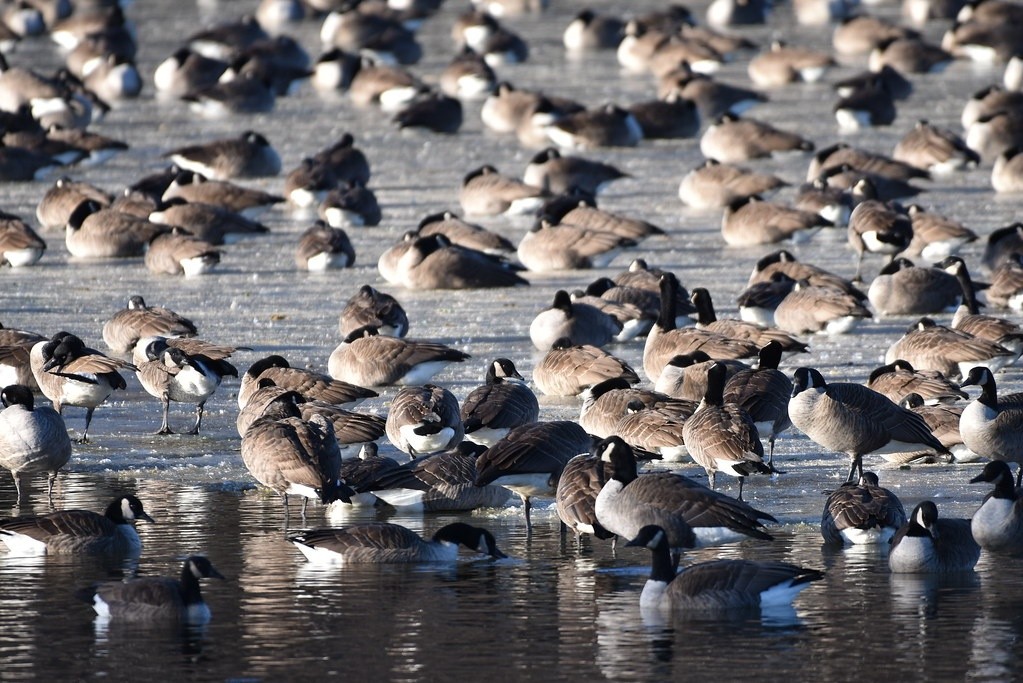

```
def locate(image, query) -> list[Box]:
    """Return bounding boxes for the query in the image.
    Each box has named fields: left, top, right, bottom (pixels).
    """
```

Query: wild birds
left=0, top=0, right=1023, bottom=619
